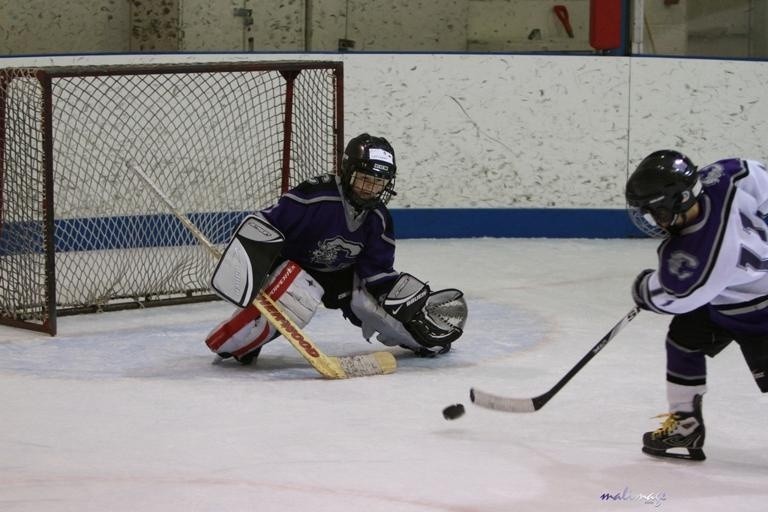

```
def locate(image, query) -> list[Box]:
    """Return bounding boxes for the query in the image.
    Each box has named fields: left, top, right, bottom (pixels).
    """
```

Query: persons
left=205, top=133, right=467, bottom=364
left=626, top=150, right=768, bottom=450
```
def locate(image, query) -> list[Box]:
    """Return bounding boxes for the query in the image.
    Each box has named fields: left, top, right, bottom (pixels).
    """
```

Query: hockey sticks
left=125, top=158, right=398, bottom=379
left=470, top=304, right=642, bottom=413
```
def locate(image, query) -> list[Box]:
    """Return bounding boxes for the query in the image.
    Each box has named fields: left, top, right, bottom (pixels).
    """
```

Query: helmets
left=340, top=133, right=397, bottom=179
left=625, top=149, right=705, bottom=213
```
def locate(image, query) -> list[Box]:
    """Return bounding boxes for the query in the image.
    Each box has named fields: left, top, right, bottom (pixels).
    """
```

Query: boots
left=643, top=412, right=706, bottom=449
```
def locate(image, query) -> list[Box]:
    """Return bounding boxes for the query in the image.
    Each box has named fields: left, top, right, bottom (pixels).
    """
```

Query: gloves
left=382, top=271, right=468, bottom=348
